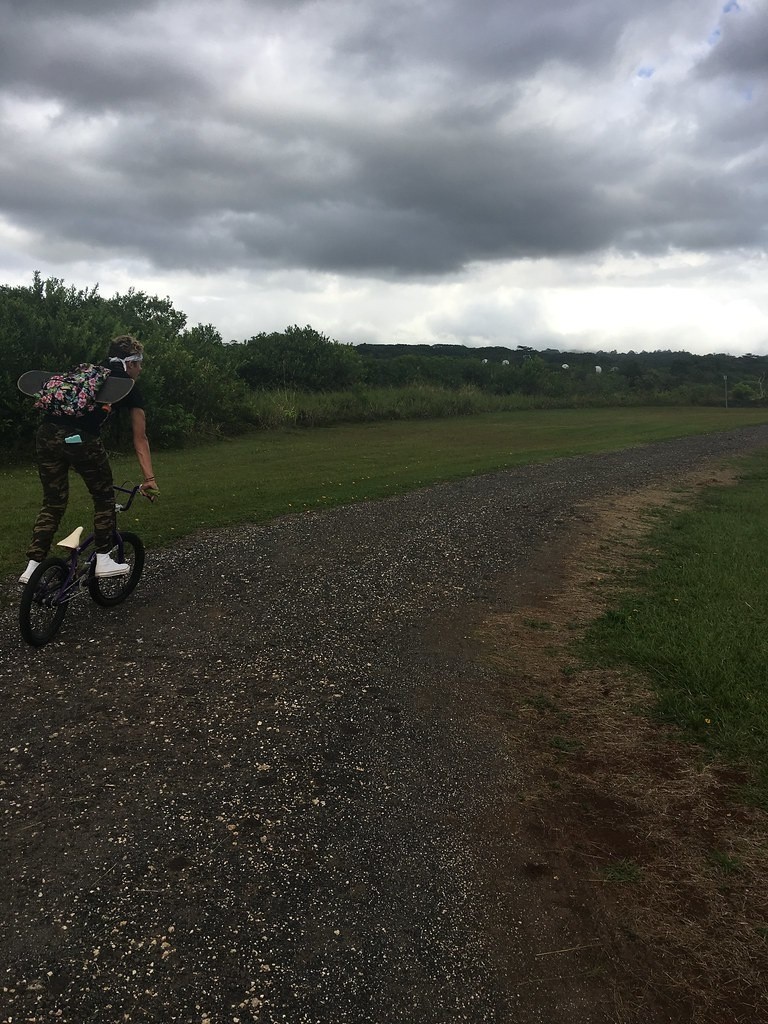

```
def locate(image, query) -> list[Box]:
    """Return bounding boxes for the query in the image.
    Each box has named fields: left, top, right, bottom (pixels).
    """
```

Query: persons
left=17, top=336, right=159, bottom=585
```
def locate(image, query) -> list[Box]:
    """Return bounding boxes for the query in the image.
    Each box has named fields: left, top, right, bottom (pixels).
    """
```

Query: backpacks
left=33, top=362, right=112, bottom=418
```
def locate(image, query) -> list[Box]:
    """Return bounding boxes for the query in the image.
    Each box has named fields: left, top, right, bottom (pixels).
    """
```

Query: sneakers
left=94, top=550, right=130, bottom=577
left=18, top=559, right=47, bottom=586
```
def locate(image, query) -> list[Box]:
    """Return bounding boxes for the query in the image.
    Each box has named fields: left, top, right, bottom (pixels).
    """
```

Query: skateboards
left=18, top=370, right=135, bottom=414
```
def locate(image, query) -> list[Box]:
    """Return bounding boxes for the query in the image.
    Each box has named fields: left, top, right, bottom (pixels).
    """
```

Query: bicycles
left=18, top=466, right=162, bottom=647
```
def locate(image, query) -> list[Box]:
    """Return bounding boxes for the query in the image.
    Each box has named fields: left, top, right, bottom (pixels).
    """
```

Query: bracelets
left=144, top=477, right=155, bottom=482
